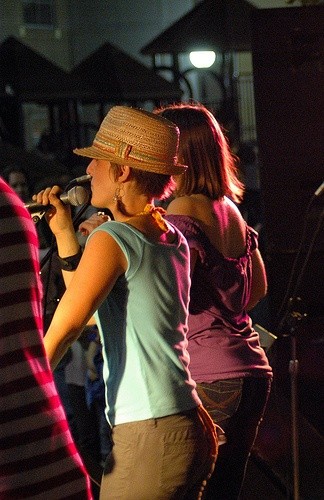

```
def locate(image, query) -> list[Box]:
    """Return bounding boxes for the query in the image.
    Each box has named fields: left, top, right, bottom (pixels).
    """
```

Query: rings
left=97, top=212, right=105, bottom=215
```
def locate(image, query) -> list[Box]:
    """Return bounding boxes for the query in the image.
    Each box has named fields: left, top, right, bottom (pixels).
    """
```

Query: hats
left=76, top=106, right=187, bottom=176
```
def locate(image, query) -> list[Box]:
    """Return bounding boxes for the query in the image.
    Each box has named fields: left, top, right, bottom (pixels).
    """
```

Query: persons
left=0, top=107, right=218, bottom=500
left=78, top=103, right=274, bottom=500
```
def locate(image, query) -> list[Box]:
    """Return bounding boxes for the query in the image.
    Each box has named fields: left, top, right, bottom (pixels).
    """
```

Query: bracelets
left=55, top=244, right=83, bottom=272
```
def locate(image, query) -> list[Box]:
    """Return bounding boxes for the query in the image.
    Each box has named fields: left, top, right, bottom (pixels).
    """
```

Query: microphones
left=76, top=175, right=91, bottom=182
left=25, top=186, right=89, bottom=212
left=314, top=182, right=324, bottom=197
